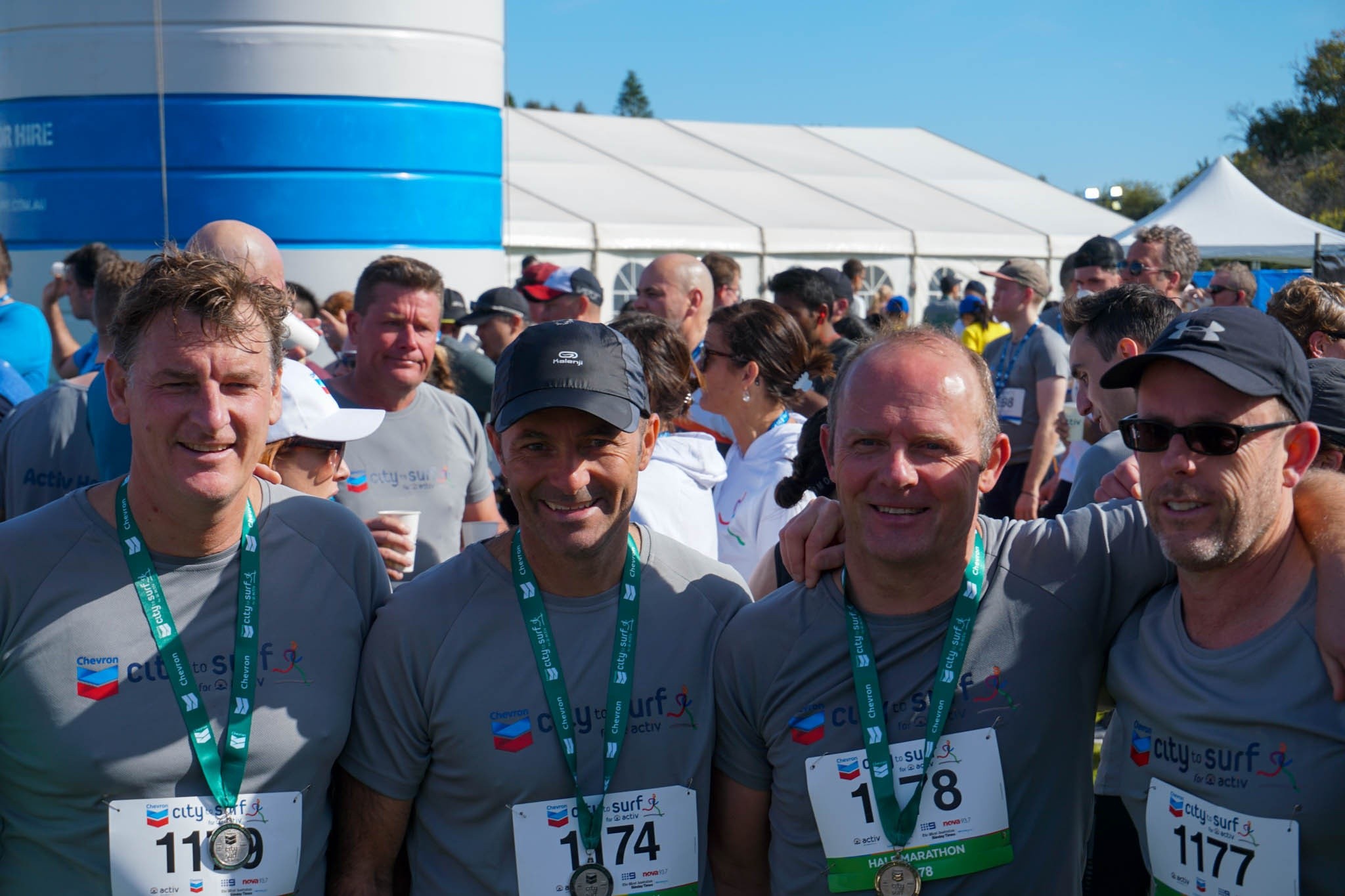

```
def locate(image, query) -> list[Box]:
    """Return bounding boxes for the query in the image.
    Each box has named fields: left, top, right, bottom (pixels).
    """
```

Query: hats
left=886, top=296, right=909, bottom=313
left=454, top=287, right=529, bottom=327
left=1072, top=236, right=1124, bottom=268
left=1097, top=306, right=1314, bottom=422
left=521, top=263, right=560, bottom=282
left=819, top=267, right=852, bottom=299
left=441, top=288, right=466, bottom=326
left=265, top=357, right=387, bottom=445
left=967, top=280, right=986, bottom=295
left=958, top=295, right=983, bottom=318
left=940, top=276, right=963, bottom=292
left=1307, top=357, right=1345, bottom=445
left=489, top=318, right=653, bottom=434
left=978, top=259, right=1051, bottom=296
left=522, top=266, right=604, bottom=306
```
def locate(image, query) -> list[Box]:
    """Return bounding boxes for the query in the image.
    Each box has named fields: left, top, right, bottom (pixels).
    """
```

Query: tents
left=1113, top=154, right=1345, bottom=267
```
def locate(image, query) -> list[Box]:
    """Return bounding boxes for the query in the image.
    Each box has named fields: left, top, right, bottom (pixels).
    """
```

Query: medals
left=875, top=847, right=921, bottom=896
left=210, top=807, right=253, bottom=870
left=570, top=848, right=614, bottom=896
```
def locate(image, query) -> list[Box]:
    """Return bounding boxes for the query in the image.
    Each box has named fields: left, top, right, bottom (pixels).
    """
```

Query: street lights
left=1110, top=185, right=1123, bottom=214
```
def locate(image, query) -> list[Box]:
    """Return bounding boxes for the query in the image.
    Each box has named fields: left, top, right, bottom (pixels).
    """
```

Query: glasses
left=726, top=286, right=741, bottom=299
left=1117, top=412, right=1300, bottom=457
left=699, top=346, right=760, bottom=387
left=1116, top=260, right=1173, bottom=277
left=1209, top=284, right=1226, bottom=294
left=469, top=301, right=524, bottom=317
left=286, top=441, right=346, bottom=472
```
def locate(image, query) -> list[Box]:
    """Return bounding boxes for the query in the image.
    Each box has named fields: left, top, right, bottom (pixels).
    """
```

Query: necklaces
left=0, top=293, right=10, bottom=303
left=767, top=409, right=789, bottom=431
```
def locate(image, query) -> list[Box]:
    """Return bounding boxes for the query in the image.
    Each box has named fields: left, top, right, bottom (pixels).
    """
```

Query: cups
left=461, top=520, right=499, bottom=548
left=279, top=308, right=321, bottom=357
left=459, top=332, right=480, bottom=352
left=1061, top=402, right=1085, bottom=442
left=378, top=510, right=422, bottom=573
left=1185, top=295, right=1215, bottom=313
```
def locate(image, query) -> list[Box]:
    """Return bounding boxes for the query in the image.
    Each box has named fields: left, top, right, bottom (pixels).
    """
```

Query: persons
left=779, top=307, right=1345, bottom=896
left=712, top=325, right=1345, bottom=896
left=44, top=241, right=121, bottom=380
left=0, top=241, right=394, bottom=895
left=328, top=318, right=756, bottom=896
left=85, top=220, right=323, bottom=482
left=0, top=238, right=52, bottom=416
left=0, top=260, right=150, bottom=515
left=288, top=224, right=1345, bottom=582
left=253, top=357, right=386, bottom=498
